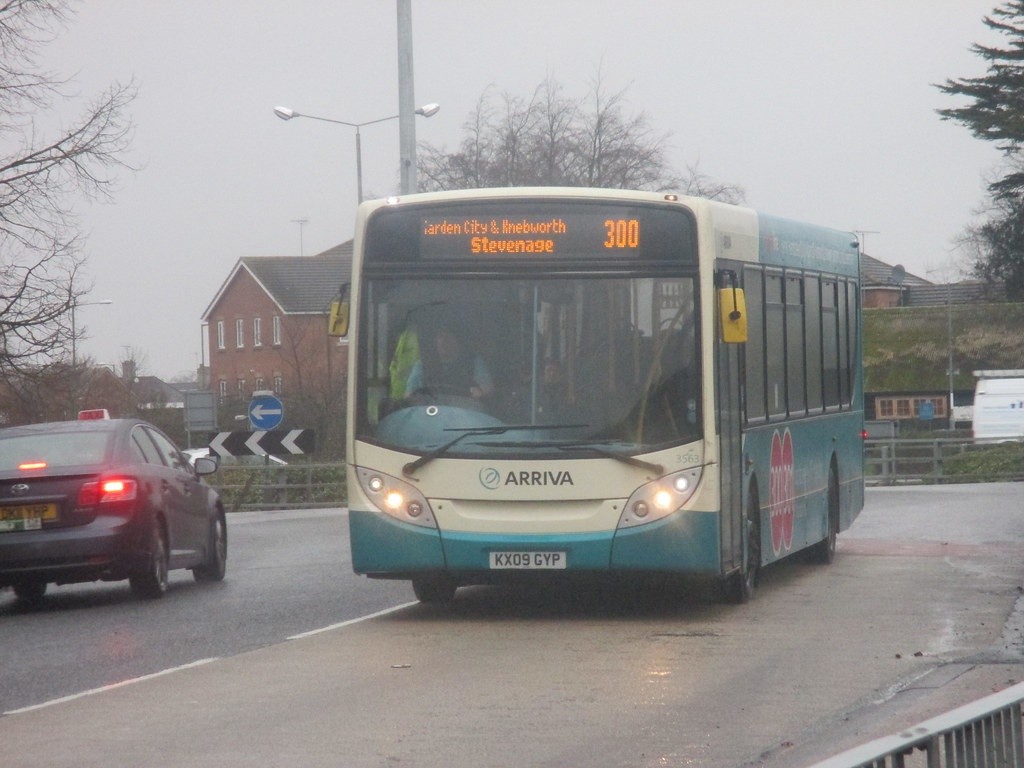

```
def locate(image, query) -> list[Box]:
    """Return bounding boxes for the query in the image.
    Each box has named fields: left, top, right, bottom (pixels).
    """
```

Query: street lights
left=272, top=102, right=441, bottom=203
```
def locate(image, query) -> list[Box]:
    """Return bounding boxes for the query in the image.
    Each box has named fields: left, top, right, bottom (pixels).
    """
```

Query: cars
left=0, top=416, right=230, bottom=604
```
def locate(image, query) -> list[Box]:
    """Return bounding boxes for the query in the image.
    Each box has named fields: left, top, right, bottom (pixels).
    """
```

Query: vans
left=972, top=376, right=1024, bottom=443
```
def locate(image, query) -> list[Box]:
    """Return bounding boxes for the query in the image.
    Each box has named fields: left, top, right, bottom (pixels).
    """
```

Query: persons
left=404, top=327, right=494, bottom=402
left=543, top=357, right=568, bottom=410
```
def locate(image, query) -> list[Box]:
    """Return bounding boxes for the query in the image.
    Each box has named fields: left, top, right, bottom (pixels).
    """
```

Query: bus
left=326, top=185, right=868, bottom=606
